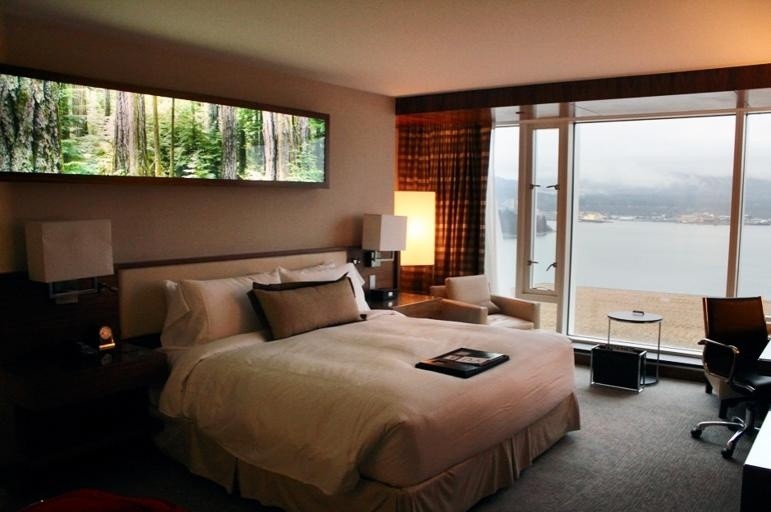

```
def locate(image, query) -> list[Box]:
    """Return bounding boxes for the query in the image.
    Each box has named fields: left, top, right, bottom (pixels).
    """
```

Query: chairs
left=430, top=285, right=541, bottom=332
left=689, top=295, right=770, bottom=457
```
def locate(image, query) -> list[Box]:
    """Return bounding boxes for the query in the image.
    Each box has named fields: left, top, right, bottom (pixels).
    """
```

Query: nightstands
left=0, top=341, right=165, bottom=511
left=367, top=288, right=442, bottom=319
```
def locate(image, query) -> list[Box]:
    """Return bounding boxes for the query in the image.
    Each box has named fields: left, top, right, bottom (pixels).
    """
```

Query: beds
left=112, top=246, right=581, bottom=511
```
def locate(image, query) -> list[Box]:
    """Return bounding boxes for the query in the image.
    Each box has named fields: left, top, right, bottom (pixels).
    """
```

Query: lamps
left=394, top=191, right=436, bottom=266
left=362, top=212, right=405, bottom=266
left=26, top=219, right=116, bottom=304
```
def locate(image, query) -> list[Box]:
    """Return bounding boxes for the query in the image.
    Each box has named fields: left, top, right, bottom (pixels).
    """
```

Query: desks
left=741, top=413, right=770, bottom=512
left=606, top=311, right=663, bottom=387
left=755, top=339, right=771, bottom=374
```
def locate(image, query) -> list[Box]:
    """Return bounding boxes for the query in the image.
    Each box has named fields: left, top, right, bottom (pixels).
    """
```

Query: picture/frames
left=0, top=63, right=330, bottom=191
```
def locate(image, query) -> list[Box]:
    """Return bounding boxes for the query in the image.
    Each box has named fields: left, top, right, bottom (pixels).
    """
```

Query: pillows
left=159, top=272, right=277, bottom=348
left=246, top=274, right=365, bottom=341
left=277, top=261, right=371, bottom=320
left=446, top=275, right=498, bottom=315
